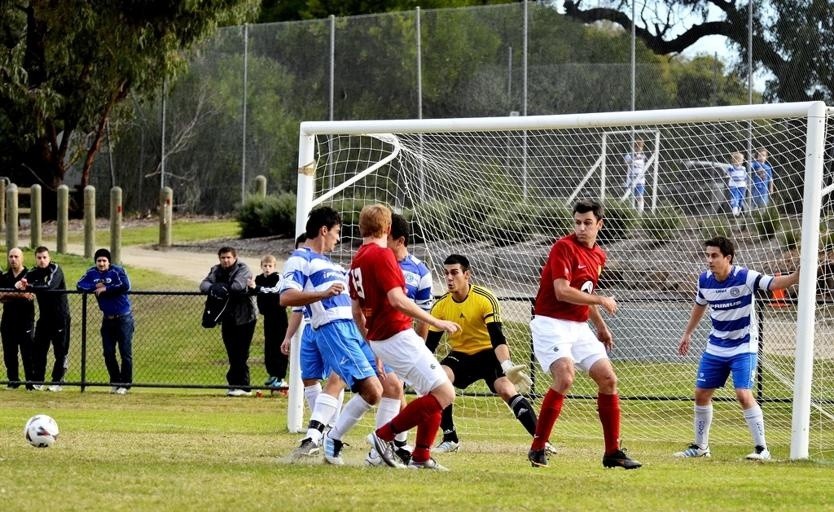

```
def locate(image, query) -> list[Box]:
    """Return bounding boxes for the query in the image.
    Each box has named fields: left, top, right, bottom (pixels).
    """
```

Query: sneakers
left=265, top=376, right=287, bottom=388
left=4, top=381, right=64, bottom=391
left=108, top=384, right=129, bottom=395
left=227, top=385, right=255, bottom=397
left=526, top=440, right=558, bottom=468
left=671, top=443, right=714, bottom=458
left=292, top=437, right=321, bottom=458
left=602, top=449, right=643, bottom=469
left=322, top=429, right=348, bottom=467
left=744, top=446, right=771, bottom=461
left=364, top=429, right=460, bottom=470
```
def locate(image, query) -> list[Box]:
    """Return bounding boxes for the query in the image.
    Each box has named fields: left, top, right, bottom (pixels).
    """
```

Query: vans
left=644, top=158, right=736, bottom=214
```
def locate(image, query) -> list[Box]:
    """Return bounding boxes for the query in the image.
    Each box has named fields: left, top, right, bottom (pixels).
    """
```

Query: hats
left=94, top=248, right=112, bottom=262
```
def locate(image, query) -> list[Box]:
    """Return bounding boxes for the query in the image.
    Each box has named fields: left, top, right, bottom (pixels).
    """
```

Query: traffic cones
left=766, top=271, right=792, bottom=307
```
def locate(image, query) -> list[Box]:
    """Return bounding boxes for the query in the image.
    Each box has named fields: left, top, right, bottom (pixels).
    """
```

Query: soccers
left=25, top=415, right=59, bottom=449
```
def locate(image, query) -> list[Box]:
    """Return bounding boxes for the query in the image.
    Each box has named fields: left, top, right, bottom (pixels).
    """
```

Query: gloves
left=498, top=358, right=533, bottom=397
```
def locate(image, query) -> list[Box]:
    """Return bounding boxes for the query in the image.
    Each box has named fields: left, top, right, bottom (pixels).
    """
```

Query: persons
left=624, top=139, right=646, bottom=216
left=76, top=249, right=135, bottom=394
left=529, top=198, right=644, bottom=469
left=14, top=246, right=72, bottom=392
left=672, top=237, right=801, bottom=459
left=424, top=254, right=556, bottom=457
left=199, top=246, right=258, bottom=397
left=725, top=153, right=747, bottom=217
left=0, top=247, right=37, bottom=391
left=278, top=201, right=464, bottom=471
left=751, top=148, right=773, bottom=216
left=247, top=255, right=289, bottom=389
